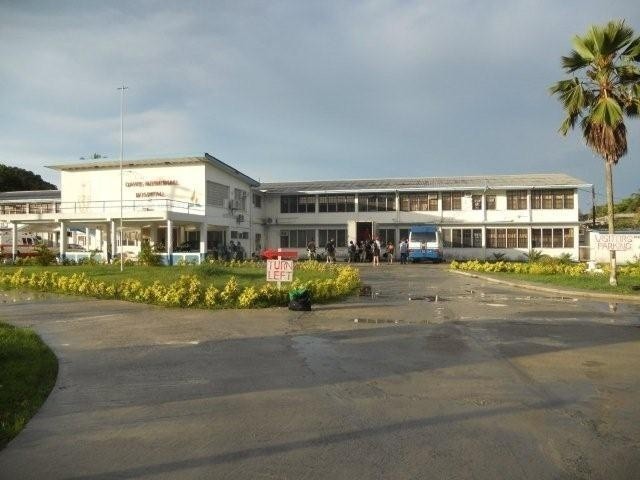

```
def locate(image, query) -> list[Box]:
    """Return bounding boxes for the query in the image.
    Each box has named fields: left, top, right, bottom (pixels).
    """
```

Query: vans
left=407, top=224, right=444, bottom=264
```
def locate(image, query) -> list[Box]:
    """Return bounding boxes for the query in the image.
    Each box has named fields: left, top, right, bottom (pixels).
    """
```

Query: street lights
left=117, top=85, right=128, bottom=271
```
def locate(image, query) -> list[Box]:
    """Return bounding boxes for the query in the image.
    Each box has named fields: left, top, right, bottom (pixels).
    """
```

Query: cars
left=174, top=240, right=218, bottom=252
left=53, top=244, right=86, bottom=251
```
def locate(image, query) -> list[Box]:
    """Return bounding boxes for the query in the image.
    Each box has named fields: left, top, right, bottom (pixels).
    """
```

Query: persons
left=228, top=240, right=236, bottom=262
left=306, top=239, right=316, bottom=261
left=355, top=242, right=363, bottom=262
left=234, top=241, right=247, bottom=263
left=359, top=232, right=409, bottom=267
left=347, top=240, right=356, bottom=263
left=254, top=238, right=262, bottom=259
left=324, top=237, right=335, bottom=264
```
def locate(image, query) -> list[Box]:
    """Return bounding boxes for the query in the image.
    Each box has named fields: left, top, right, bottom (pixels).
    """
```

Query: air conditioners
left=239, top=215, right=244, bottom=222
left=266, top=217, right=275, bottom=224
left=230, top=200, right=241, bottom=209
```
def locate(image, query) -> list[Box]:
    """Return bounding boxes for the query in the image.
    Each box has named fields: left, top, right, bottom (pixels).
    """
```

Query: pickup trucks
left=0, top=235, right=44, bottom=259
